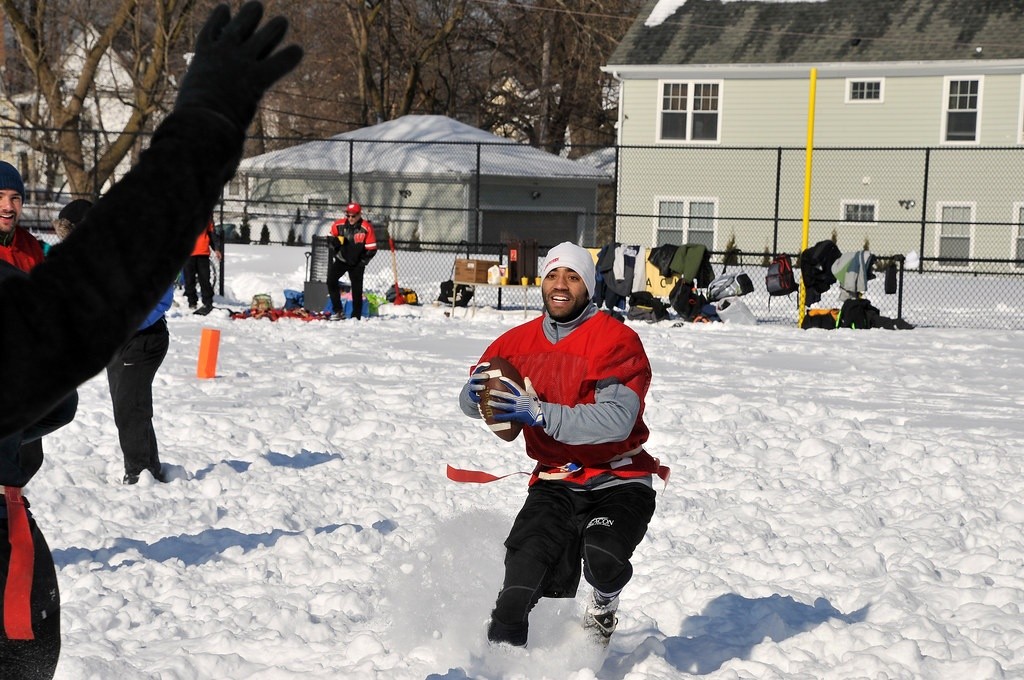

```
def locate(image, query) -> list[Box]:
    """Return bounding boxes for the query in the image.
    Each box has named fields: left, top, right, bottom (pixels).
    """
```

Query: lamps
left=898, top=200, right=915, bottom=210
left=532, top=191, right=541, bottom=199
left=399, top=190, right=411, bottom=198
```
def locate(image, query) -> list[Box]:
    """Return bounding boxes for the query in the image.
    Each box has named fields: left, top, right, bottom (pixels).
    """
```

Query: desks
left=452, top=281, right=540, bottom=319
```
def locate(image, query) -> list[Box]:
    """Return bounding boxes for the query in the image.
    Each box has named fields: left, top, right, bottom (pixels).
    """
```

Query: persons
left=183, top=218, right=221, bottom=310
left=0, top=0, right=304, bottom=679
left=0, top=161, right=43, bottom=272
left=330, top=203, right=377, bottom=322
left=38, top=199, right=174, bottom=484
left=459, top=241, right=656, bottom=670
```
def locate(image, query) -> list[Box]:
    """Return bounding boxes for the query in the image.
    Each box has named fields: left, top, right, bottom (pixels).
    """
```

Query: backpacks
left=766, top=252, right=799, bottom=297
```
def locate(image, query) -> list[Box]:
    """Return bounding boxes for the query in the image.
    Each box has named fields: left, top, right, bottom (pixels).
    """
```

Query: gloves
left=487, top=376, right=545, bottom=427
left=469, top=362, right=491, bottom=403
left=175, top=0, right=304, bottom=134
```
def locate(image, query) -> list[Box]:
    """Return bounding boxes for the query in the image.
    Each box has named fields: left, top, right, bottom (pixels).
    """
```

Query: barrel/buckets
left=487, top=264, right=501, bottom=284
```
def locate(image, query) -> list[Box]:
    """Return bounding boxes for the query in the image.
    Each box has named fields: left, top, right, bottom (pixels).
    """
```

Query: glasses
left=346, top=214, right=360, bottom=218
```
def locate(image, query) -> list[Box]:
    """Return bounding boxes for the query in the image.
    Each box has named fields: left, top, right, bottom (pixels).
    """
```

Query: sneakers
left=330, top=311, right=346, bottom=321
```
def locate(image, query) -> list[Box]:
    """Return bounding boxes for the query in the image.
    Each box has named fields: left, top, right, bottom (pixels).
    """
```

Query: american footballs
left=476, top=355, right=527, bottom=443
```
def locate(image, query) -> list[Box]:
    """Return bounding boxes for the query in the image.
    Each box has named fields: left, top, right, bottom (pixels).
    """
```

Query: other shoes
left=580, top=595, right=617, bottom=670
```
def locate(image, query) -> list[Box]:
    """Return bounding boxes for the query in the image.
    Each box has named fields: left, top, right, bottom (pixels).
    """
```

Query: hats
left=541, top=242, right=595, bottom=300
left=58, top=199, right=94, bottom=227
left=345, top=204, right=361, bottom=213
left=0, top=161, right=25, bottom=205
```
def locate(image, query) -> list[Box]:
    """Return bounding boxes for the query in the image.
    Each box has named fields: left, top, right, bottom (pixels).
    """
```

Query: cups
left=535, top=277, right=541, bottom=286
left=500, top=277, right=506, bottom=285
left=522, top=278, right=527, bottom=286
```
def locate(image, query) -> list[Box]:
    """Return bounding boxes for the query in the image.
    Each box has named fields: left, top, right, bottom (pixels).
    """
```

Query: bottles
left=692, top=287, right=698, bottom=293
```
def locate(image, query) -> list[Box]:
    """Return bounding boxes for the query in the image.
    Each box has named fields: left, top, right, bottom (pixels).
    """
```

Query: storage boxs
left=454, top=260, right=500, bottom=283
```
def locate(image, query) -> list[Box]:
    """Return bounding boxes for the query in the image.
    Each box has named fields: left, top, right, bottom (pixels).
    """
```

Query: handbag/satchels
left=839, top=298, right=878, bottom=329
left=707, top=248, right=755, bottom=301
left=668, top=278, right=710, bottom=319
left=802, top=308, right=840, bottom=330
left=715, top=297, right=757, bottom=326
